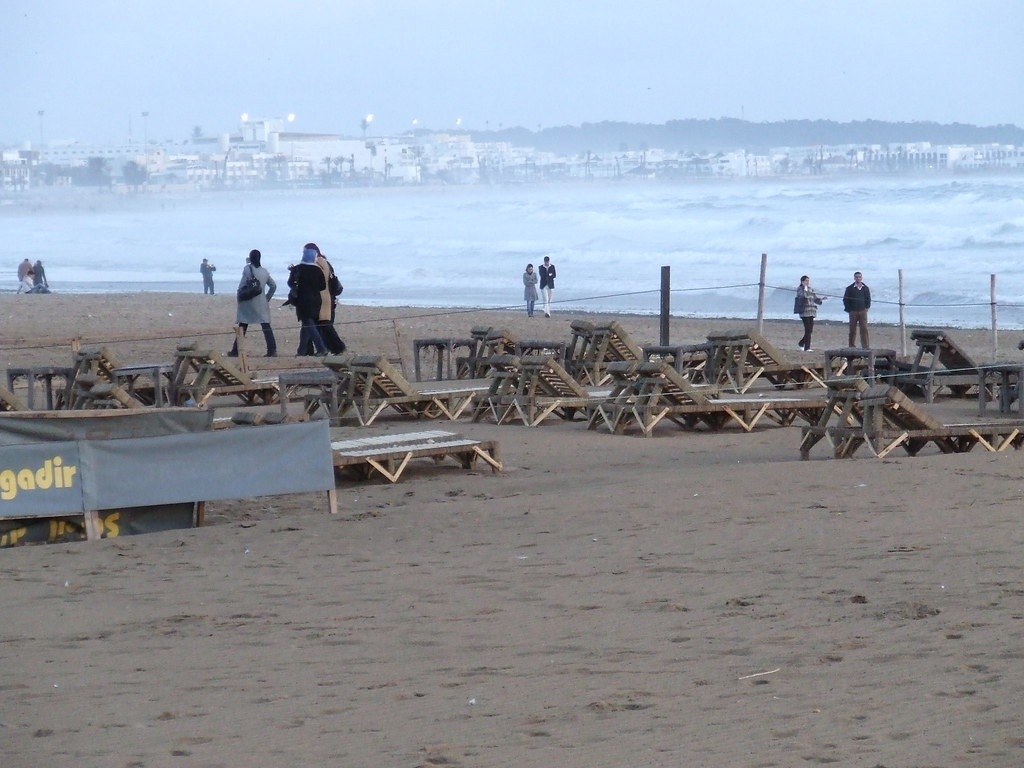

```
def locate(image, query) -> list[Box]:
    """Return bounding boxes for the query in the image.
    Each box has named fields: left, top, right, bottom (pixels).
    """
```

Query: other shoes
left=228, top=348, right=347, bottom=357
left=807, top=349, right=814, bottom=352
left=797, top=345, right=804, bottom=351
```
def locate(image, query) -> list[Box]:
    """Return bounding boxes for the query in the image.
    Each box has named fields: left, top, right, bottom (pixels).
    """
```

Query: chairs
left=0, top=324, right=1024, bottom=485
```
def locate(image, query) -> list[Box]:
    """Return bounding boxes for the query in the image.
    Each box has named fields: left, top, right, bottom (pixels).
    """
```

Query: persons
left=228, top=250, right=276, bottom=358
left=797, top=276, right=828, bottom=353
left=842, top=272, right=872, bottom=349
left=524, top=263, right=538, bottom=317
left=282, top=244, right=348, bottom=356
left=15, top=258, right=50, bottom=294
left=538, top=256, right=556, bottom=317
left=201, top=257, right=217, bottom=294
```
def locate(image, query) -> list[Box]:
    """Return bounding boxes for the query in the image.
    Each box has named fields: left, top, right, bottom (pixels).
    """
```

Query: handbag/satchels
left=794, top=297, right=808, bottom=314
left=288, top=266, right=300, bottom=304
left=331, top=274, right=343, bottom=296
left=239, top=265, right=262, bottom=301
